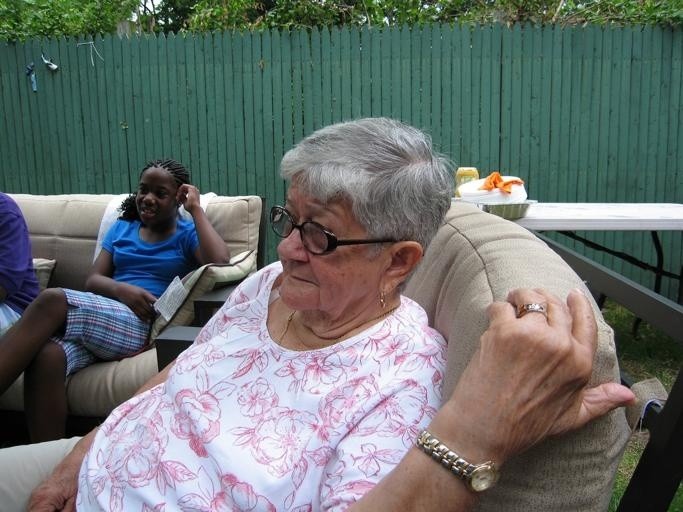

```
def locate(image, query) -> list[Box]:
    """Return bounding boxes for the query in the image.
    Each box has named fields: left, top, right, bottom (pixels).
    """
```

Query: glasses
left=267, top=204, right=400, bottom=256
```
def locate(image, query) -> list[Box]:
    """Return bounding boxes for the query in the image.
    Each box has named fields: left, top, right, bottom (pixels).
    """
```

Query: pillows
left=139, top=248, right=258, bottom=352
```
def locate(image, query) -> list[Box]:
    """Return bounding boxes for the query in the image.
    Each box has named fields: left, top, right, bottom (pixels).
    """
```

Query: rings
left=516, top=302, right=548, bottom=319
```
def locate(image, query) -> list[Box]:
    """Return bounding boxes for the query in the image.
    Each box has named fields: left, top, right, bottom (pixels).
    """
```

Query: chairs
left=400, top=203, right=633, bottom=512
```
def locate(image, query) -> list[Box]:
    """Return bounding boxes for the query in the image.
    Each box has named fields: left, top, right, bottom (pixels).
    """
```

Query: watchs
left=416, top=429, right=499, bottom=492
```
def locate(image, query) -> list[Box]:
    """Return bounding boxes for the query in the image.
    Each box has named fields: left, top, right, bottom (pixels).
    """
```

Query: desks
left=514, top=198, right=682, bottom=354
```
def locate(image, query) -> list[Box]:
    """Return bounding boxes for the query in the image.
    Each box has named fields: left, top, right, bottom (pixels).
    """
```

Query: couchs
left=1, top=190, right=266, bottom=428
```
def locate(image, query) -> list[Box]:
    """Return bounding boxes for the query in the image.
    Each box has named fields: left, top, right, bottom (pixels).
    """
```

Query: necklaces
left=278, top=302, right=402, bottom=345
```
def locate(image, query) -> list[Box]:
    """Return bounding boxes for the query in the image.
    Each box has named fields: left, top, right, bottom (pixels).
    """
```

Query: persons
left=23, top=116, right=639, bottom=511
left=1, top=158, right=231, bottom=445
left=0, top=191, right=41, bottom=340
